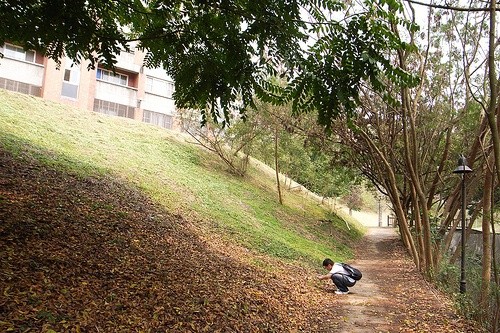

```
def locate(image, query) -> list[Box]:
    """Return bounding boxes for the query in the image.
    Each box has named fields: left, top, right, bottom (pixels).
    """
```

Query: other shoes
left=334, top=289, right=349, bottom=294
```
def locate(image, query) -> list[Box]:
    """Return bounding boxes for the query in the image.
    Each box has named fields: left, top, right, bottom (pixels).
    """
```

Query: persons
left=317, top=259, right=356, bottom=295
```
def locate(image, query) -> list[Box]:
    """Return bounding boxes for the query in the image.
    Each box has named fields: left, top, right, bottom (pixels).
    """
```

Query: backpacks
left=338, top=263, right=362, bottom=281
